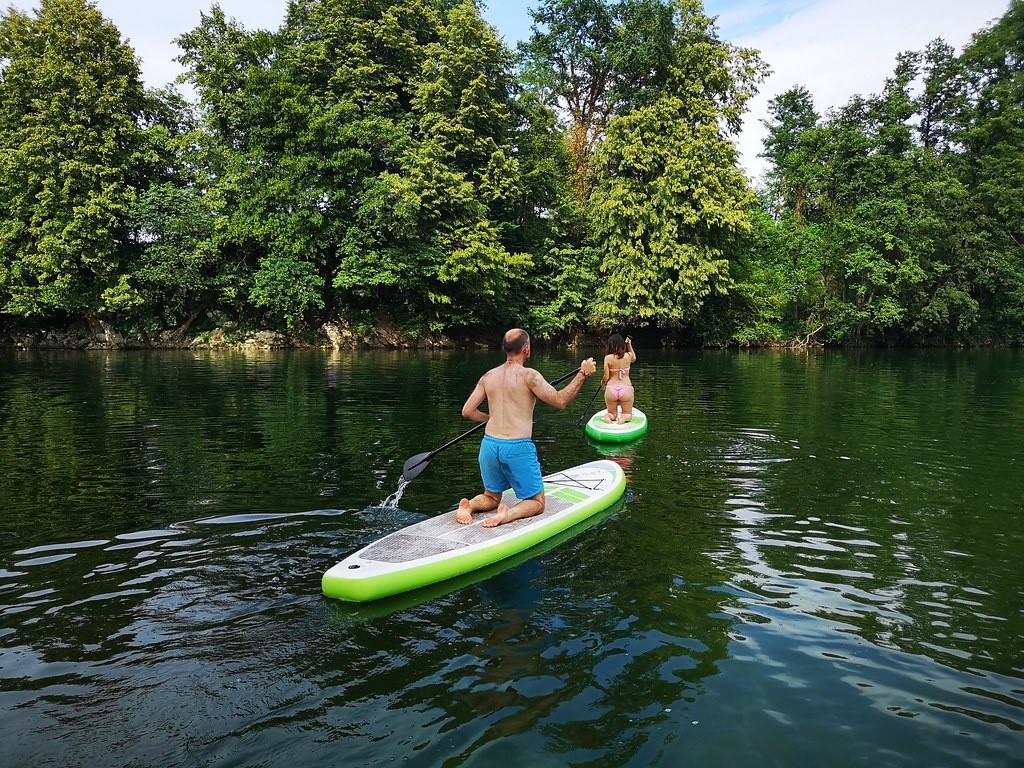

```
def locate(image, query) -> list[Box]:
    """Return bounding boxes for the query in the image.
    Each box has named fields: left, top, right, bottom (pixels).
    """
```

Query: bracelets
left=578, top=370, right=589, bottom=379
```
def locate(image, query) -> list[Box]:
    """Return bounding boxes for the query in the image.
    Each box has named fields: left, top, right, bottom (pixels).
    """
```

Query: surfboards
left=317, top=457, right=629, bottom=606
left=584, top=404, right=649, bottom=444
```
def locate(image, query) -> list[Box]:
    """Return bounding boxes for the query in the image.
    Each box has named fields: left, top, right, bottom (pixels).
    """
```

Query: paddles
left=575, top=334, right=634, bottom=428
left=401, top=366, right=581, bottom=482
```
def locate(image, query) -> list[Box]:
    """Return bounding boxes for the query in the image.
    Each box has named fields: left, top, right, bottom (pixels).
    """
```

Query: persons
left=456, top=329, right=596, bottom=528
left=601, top=335, right=636, bottom=424
